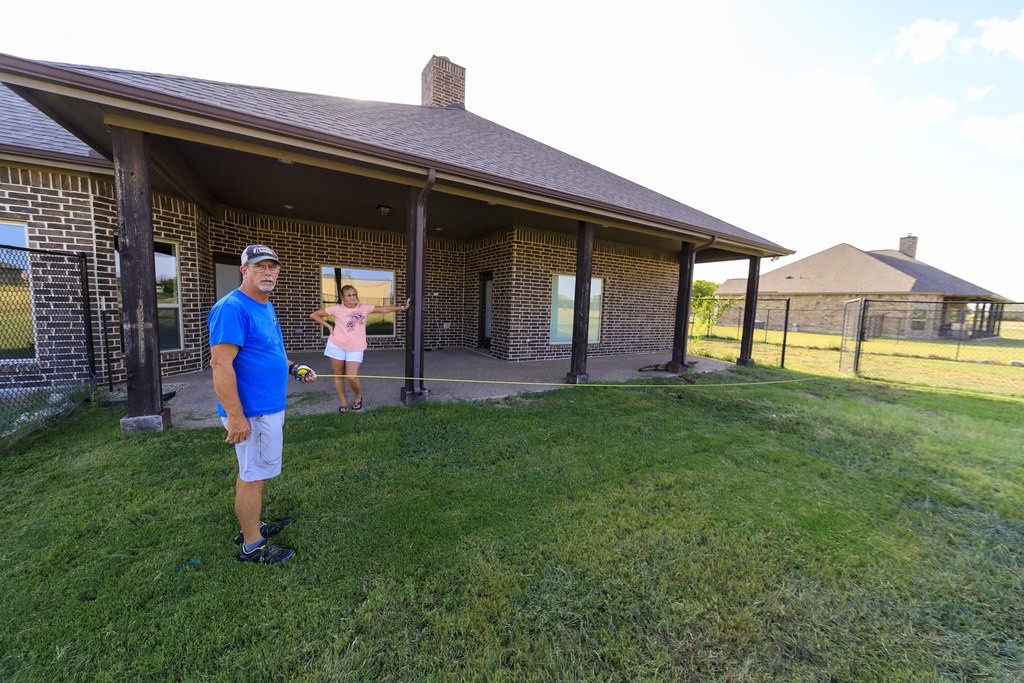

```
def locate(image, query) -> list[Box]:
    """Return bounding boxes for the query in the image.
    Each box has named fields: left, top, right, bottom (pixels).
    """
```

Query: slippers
left=339, top=402, right=350, bottom=413
left=351, top=397, right=362, bottom=410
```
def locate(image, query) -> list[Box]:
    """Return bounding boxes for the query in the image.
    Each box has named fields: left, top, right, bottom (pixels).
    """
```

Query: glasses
left=246, top=264, right=279, bottom=273
left=347, top=294, right=356, bottom=297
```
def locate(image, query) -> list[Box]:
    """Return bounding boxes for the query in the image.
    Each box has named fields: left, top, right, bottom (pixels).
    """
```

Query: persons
left=310, top=284, right=411, bottom=413
left=207, top=243, right=317, bottom=565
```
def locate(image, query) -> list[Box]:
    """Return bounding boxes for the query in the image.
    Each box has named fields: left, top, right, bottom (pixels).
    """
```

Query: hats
left=240, top=244, right=283, bottom=268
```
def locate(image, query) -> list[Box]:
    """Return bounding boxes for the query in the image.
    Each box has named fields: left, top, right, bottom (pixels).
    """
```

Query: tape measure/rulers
left=297, top=364, right=822, bottom=388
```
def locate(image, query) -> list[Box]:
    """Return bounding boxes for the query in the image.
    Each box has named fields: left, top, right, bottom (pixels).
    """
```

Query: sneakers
left=238, top=538, right=297, bottom=564
left=233, top=518, right=293, bottom=545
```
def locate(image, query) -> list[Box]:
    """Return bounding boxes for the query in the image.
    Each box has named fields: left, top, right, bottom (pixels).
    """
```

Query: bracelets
left=289, top=362, right=295, bottom=374
left=401, top=305, right=405, bottom=311
